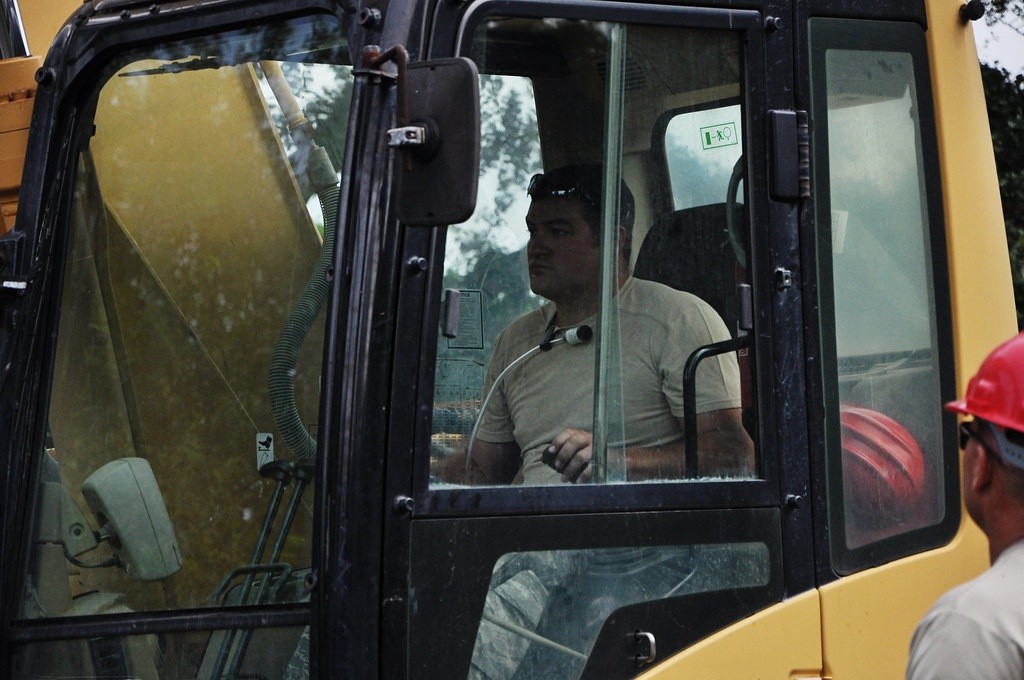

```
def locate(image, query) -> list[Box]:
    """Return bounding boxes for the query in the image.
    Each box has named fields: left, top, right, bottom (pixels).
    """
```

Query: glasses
left=958, top=421, right=1004, bottom=469
left=527, top=173, right=599, bottom=210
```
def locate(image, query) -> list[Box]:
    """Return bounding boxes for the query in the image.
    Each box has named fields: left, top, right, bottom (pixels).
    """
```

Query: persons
left=907, top=333, right=1024, bottom=680
left=289, top=164, right=757, bottom=680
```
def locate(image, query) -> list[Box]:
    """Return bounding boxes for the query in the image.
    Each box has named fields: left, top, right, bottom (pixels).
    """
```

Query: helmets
left=840, top=403, right=924, bottom=518
left=943, top=330, right=1024, bottom=433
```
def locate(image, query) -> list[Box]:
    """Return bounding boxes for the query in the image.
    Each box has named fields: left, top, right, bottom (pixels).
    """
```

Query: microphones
left=465, top=326, right=592, bottom=484
